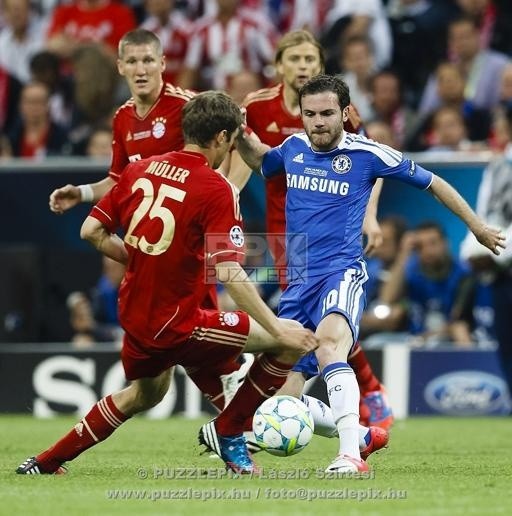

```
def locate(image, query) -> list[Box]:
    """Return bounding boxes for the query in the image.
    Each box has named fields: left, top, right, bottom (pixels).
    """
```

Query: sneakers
left=15, top=456, right=68, bottom=474
left=365, top=382, right=393, bottom=428
left=360, top=425, right=389, bottom=461
left=200, top=419, right=262, bottom=474
left=326, top=454, right=368, bottom=472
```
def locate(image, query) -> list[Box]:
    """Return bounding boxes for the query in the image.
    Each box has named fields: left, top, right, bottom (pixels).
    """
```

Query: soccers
left=252, top=396, right=315, bottom=457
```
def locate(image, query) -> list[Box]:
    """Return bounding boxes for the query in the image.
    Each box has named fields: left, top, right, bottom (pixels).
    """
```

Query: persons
left=1, top=0, right=512, bottom=475
